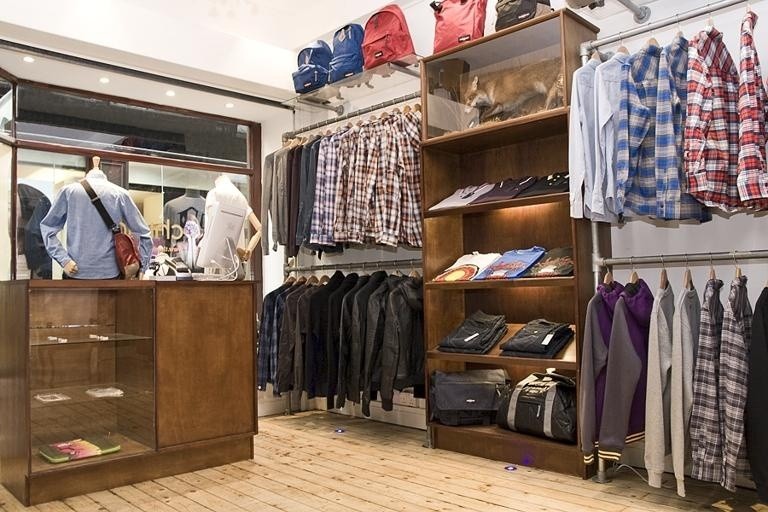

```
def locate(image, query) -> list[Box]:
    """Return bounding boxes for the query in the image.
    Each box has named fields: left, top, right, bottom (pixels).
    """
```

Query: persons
left=39, top=168, right=154, bottom=281
left=162, top=183, right=210, bottom=244
left=195, top=175, right=263, bottom=281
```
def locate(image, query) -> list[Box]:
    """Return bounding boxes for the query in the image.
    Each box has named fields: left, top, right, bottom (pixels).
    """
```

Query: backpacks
left=327, top=23, right=373, bottom=87
left=429, top=0, right=486, bottom=53
left=291, top=39, right=343, bottom=104
left=495, top=0, right=554, bottom=31
left=360, top=3, right=418, bottom=77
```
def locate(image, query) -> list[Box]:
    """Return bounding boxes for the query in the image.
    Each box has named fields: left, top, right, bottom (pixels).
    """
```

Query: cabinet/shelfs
left=2, top=279, right=259, bottom=508
left=416, top=9, right=613, bottom=480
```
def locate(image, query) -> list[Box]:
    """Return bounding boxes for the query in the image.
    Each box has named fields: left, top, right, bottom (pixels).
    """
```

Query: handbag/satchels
left=427, top=368, right=511, bottom=427
left=112, top=232, right=143, bottom=279
left=495, top=371, right=576, bottom=443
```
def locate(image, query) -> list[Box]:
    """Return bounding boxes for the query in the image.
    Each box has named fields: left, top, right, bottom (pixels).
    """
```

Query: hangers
left=282, top=92, right=421, bottom=150
left=591, top=1, right=752, bottom=62
left=598, top=249, right=744, bottom=288
left=285, top=258, right=418, bottom=285
